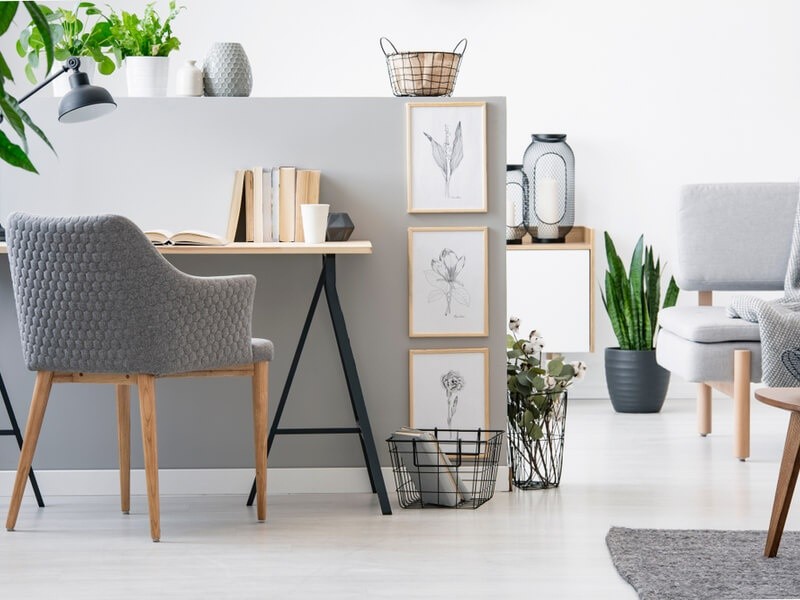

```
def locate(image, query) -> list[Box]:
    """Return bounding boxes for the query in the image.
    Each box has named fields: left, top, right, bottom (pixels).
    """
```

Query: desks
left=0, top=240, right=391, bottom=516
left=754, top=387, right=800, bottom=559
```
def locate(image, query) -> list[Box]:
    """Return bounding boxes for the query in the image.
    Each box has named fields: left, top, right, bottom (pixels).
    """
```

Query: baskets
left=386, top=427, right=505, bottom=510
left=379, top=36, right=468, bottom=97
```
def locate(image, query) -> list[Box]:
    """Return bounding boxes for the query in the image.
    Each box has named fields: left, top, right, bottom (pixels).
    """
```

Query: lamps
left=0, top=55, right=118, bottom=241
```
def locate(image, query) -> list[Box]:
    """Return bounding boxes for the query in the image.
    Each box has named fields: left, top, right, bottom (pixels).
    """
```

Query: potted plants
left=16, top=0, right=115, bottom=97
left=597, top=232, right=680, bottom=413
left=86, top=0, right=186, bottom=95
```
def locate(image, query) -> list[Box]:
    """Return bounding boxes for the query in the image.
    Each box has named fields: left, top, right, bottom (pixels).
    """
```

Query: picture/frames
left=407, top=101, right=488, bottom=213
left=408, top=348, right=490, bottom=458
left=407, top=227, right=490, bottom=338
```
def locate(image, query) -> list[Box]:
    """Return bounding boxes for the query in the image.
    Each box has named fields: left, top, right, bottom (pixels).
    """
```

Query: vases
left=176, top=60, right=204, bottom=97
left=205, top=42, right=253, bottom=96
left=506, top=389, right=568, bottom=488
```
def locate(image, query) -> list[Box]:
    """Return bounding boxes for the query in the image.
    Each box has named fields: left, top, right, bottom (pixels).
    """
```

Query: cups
left=300, top=204, right=331, bottom=243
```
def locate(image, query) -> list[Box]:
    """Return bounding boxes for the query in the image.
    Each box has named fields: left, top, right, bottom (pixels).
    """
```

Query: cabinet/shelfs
left=506, top=226, right=597, bottom=362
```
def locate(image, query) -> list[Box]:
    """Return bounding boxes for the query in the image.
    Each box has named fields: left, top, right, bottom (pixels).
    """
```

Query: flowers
left=499, top=315, right=587, bottom=484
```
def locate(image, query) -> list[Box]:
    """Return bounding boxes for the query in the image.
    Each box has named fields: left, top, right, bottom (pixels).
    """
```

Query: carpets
left=606, top=527, right=800, bottom=600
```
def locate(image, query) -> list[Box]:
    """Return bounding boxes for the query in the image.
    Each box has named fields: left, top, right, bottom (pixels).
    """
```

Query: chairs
left=5, top=210, right=274, bottom=542
left=654, top=181, right=800, bottom=469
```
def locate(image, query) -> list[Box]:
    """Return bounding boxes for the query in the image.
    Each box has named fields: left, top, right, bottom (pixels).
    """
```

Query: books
left=390, top=426, right=471, bottom=506
left=143, top=232, right=222, bottom=246
left=226, top=171, right=245, bottom=242
left=245, top=166, right=321, bottom=243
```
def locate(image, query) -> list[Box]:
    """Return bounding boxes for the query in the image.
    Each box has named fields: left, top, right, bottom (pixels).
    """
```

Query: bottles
left=177, top=60, right=203, bottom=96
left=202, top=42, right=253, bottom=97
